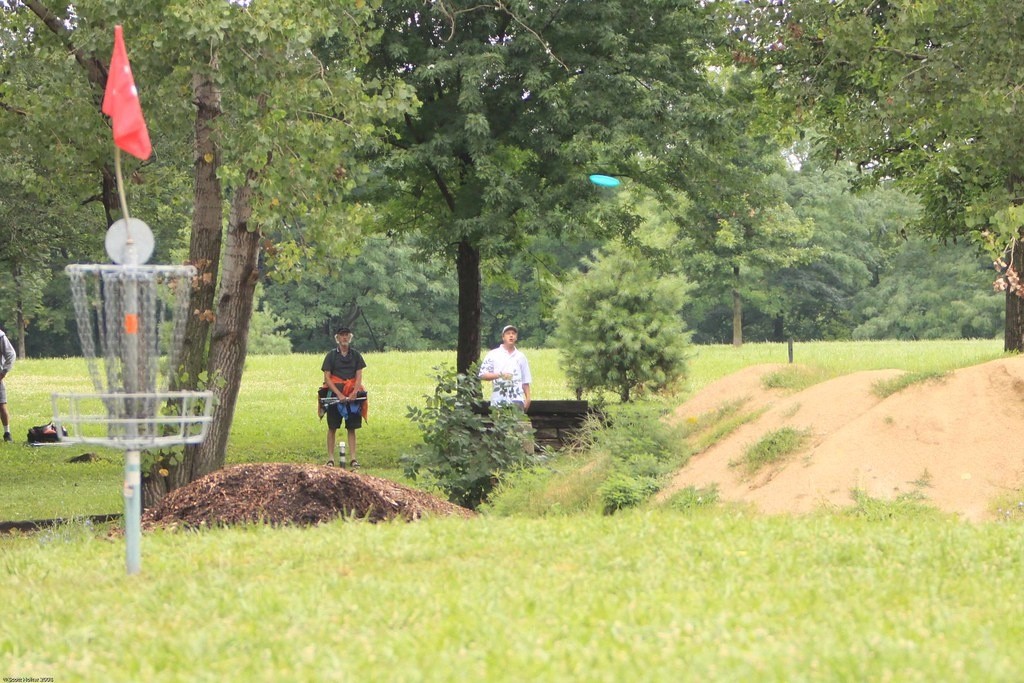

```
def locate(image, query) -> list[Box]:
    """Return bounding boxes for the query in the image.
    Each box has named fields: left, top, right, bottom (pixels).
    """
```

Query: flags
left=102, top=24, right=153, bottom=161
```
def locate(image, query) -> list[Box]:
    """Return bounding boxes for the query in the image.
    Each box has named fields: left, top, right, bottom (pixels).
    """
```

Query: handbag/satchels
left=28, top=421, right=69, bottom=444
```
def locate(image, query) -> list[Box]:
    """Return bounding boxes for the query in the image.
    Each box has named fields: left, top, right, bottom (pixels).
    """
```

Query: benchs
left=457, top=401, right=589, bottom=449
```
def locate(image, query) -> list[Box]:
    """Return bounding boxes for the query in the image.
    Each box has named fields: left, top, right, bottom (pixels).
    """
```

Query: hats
left=503, top=325, right=518, bottom=335
left=337, top=327, right=351, bottom=333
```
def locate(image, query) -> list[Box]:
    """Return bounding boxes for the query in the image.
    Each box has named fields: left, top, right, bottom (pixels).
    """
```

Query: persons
left=321, top=327, right=366, bottom=469
left=479, top=324, right=535, bottom=456
left=0, top=330, right=16, bottom=443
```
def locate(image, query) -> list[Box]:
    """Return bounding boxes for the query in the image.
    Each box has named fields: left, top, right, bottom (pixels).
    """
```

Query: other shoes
left=327, top=461, right=334, bottom=467
left=4, top=432, right=12, bottom=442
left=350, top=460, right=360, bottom=469
